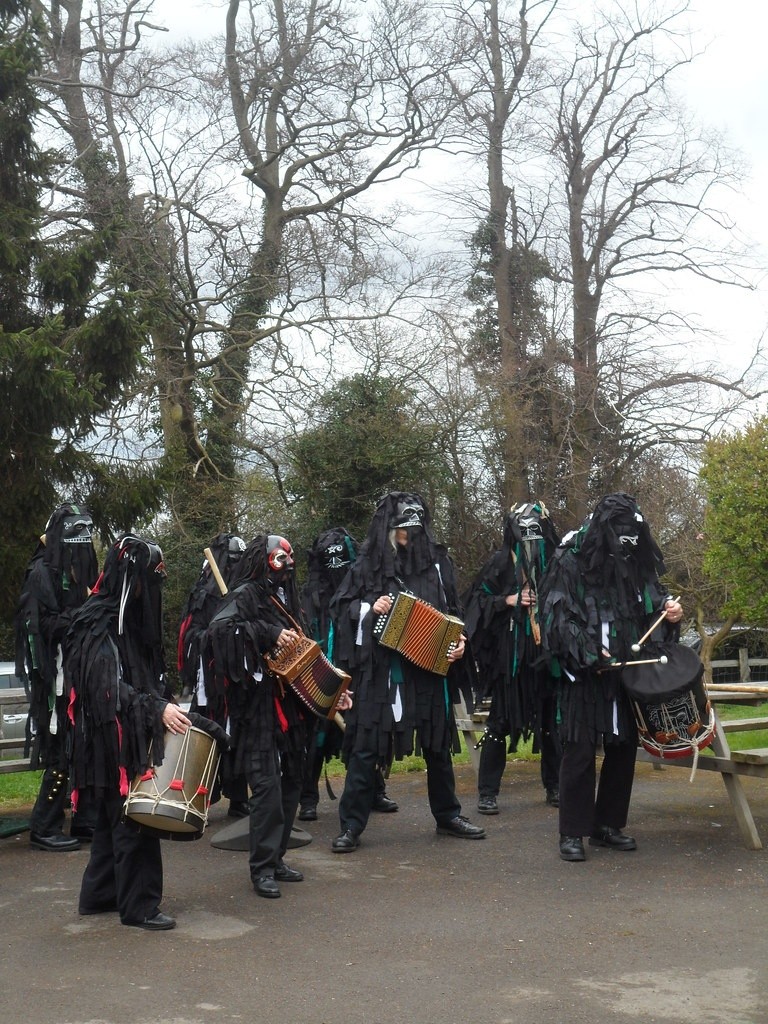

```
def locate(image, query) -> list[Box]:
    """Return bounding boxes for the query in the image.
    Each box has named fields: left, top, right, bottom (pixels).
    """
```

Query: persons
left=204, top=534, right=353, bottom=898
left=299, top=526, right=401, bottom=821
left=327, top=492, right=488, bottom=853
left=15, top=503, right=100, bottom=852
left=177, top=533, right=246, bottom=820
left=62, top=533, right=193, bottom=930
left=462, top=501, right=563, bottom=815
left=540, top=491, right=682, bottom=862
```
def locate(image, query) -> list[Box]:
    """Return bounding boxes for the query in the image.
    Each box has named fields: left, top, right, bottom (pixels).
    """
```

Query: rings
left=169, top=722, right=174, bottom=727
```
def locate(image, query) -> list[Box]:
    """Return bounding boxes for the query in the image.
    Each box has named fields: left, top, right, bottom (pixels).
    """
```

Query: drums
left=122, top=712, right=235, bottom=843
left=620, top=640, right=718, bottom=759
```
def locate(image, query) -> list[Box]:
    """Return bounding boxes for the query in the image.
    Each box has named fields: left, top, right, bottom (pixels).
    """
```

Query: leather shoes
left=559, top=834, right=585, bottom=860
left=122, top=912, right=176, bottom=930
left=436, top=815, right=486, bottom=838
left=299, top=803, right=316, bottom=820
left=254, top=876, right=279, bottom=896
left=228, top=800, right=251, bottom=818
left=588, top=824, right=636, bottom=850
left=477, top=796, right=499, bottom=813
left=546, top=788, right=560, bottom=805
left=372, top=796, right=398, bottom=811
left=332, top=826, right=360, bottom=851
left=70, top=826, right=95, bottom=839
left=29, top=831, right=80, bottom=851
left=274, top=864, right=302, bottom=881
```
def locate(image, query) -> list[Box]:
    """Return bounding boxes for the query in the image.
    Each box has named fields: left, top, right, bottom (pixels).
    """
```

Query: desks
left=634, top=679, right=768, bottom=850
left=0, top=686, right=31, bottom=757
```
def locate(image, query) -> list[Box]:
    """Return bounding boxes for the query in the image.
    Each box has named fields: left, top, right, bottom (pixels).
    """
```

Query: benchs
left=0, top=759, right=44, bottom=774
left=720, top=717, right=768, bottom=733
left=471, top=711, right=490, bottom=723
left=731, top=747, right=768, bottom=764
left=0, top=738, right=36, bottom=749
